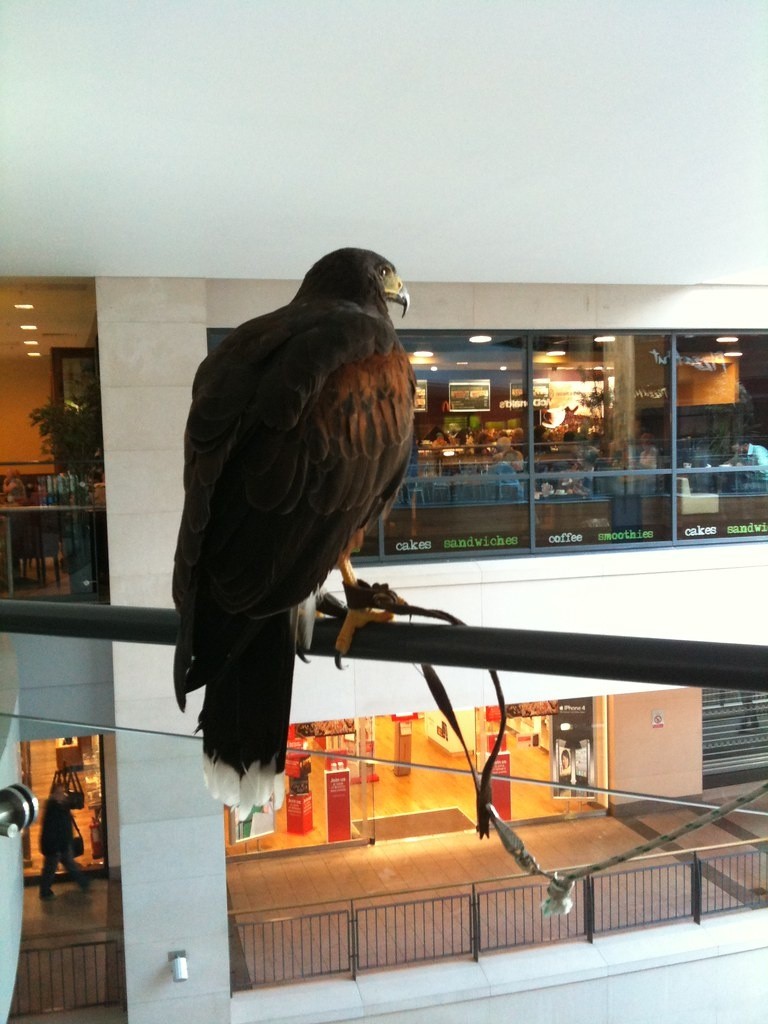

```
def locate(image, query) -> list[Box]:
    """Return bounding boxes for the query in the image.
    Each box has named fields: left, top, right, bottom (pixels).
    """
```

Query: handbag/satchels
left=72, top=814, right=85, bottom=859
left=54, top=767, right=85, bottom=809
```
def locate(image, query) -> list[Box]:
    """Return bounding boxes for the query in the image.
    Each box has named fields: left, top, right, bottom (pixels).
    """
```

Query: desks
left=54, top=736, right=84, bottom=771
left=0, top=505, right=87, bottom=589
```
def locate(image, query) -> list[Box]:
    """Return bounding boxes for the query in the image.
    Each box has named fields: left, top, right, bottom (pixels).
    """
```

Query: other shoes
left=78, top=872, right=98, bottom=888
left=41, top=891, right=57, bottom=902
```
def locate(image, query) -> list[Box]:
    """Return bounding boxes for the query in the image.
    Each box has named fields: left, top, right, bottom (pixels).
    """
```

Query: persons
left=36, top=782, right=91, bottom=899
left=560, top=749, right=572, bottom=776
left=721, top=436, right=768, bottom=492
left=428, top=426, right=663, bottom=500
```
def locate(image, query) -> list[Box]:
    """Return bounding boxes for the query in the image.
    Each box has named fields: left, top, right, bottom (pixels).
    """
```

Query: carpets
left=351, top=806, right=476, bottom=844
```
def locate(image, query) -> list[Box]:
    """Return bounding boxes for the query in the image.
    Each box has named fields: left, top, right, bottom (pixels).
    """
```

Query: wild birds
left=168, top=246, right=412, bottom=824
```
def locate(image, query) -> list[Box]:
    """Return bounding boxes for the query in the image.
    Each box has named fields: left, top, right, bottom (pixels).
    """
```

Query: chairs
left=405, top=464, right=494, bottom=505
left=677, top=477, right=719, bottom=535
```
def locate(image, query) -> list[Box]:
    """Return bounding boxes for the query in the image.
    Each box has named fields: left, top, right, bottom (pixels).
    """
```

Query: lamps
left=168, top=950, right=189, bottom=982
